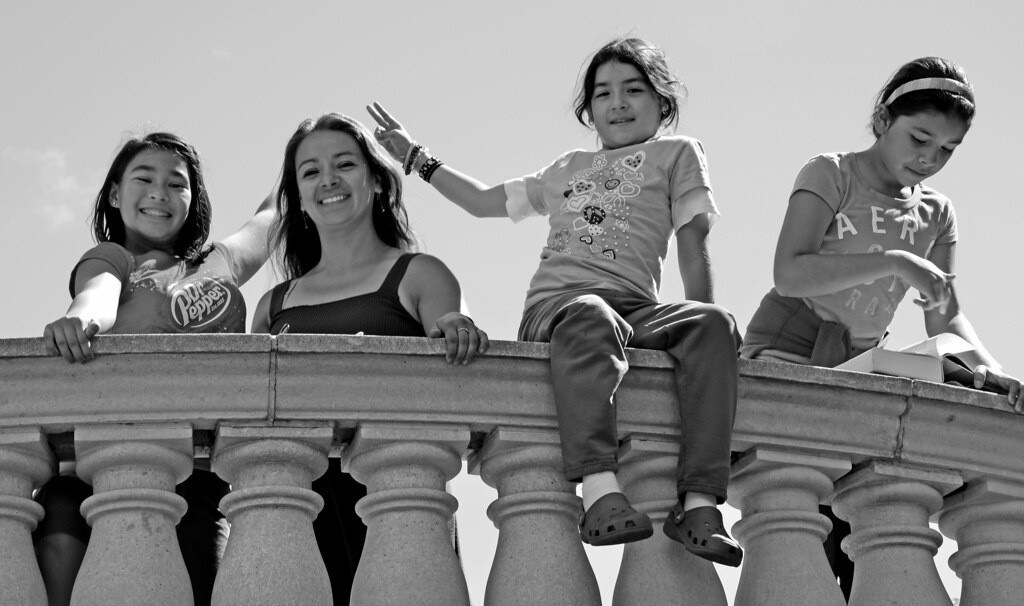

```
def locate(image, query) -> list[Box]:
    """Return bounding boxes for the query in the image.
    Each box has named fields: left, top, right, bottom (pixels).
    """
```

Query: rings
left=458, top=327, right=469, bottom=333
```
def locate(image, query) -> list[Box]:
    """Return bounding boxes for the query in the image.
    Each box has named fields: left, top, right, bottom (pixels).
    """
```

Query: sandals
left=662, top=499, right=743, bottom=568
left=577, top=492, right=654, bottom=547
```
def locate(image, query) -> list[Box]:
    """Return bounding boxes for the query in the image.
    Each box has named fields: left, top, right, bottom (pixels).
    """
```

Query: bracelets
left=401, top=141, right=425, bottom=176
left=418, top=157, right=444, bottom=184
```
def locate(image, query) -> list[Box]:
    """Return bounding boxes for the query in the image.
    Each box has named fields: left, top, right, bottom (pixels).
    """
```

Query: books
left=831, top=331, right=1017, bottom=399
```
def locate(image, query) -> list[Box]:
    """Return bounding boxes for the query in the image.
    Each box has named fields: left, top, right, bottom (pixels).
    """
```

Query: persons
left=366, top=37, right=743, bottom=567
left=251, top=111, right=490, bottom=606
left=737, top=57, right=1024, bottom=604
left=32, top=132, right=296, bottom=606
